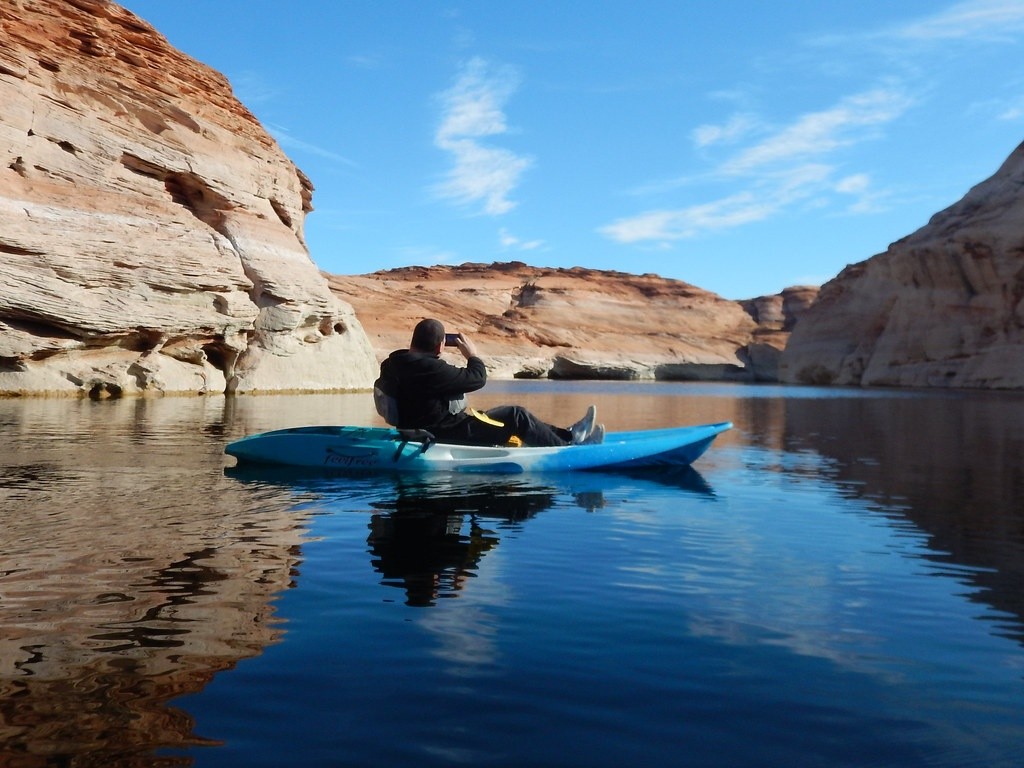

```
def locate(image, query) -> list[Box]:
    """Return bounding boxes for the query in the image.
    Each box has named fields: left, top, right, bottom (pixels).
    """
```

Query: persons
left=380, top=318, right=605, bottom=448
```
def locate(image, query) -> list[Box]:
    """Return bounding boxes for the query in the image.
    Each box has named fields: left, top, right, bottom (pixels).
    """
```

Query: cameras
left=445, top=334, right=461, bottom=346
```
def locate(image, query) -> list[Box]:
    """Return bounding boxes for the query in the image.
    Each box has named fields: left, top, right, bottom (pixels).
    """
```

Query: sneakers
left=571, top=405, right=596, bottom=441
left=579, top=426, right=604, bottom=444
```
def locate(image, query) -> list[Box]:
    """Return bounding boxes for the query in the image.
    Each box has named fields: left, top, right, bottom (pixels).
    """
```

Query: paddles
left=470, top=408, right=504, bottom=428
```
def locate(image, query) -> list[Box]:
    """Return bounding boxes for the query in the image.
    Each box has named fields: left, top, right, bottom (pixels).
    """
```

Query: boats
left=223, top=424, right=733, bottom=475
